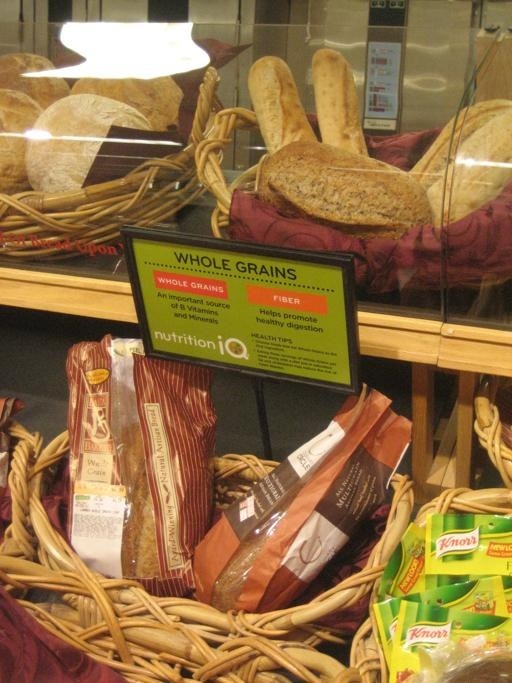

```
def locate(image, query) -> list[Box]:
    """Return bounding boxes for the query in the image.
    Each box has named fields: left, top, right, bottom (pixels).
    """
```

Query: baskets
left=2, top=420, right=414, bottom=636
left=347, top=483, right=512, bottom=683
left=473, top=375, right=512, bottom=486
left=1, top=599, right=361, bottom=683
left=1, top=65, right=225, bottom=264
left=196, top=104, right=512, bottom=294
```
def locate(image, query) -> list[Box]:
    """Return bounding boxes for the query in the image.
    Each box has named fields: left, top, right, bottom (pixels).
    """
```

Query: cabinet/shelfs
left=0, top=167, right=512, bottom=517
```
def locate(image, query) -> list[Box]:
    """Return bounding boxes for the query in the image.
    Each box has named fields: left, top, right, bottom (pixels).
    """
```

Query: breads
left=0, top=86, right=43, bottom=195
left=26, top=92, right=151, bottom=196
left=247, top=56, right=320, bottom=154
left=409, top=98, right=508, bottom=228
left=309, top=49, right=371, bottom=157
left=0, top=50, right=71, bottom=109
left=256, top=137, right=433, bottom=239
left=114, top=420, right=163, bottom=580
left=68, top=74, right=184, bottom=132
left=210, top=484, right=308, bottom=612
